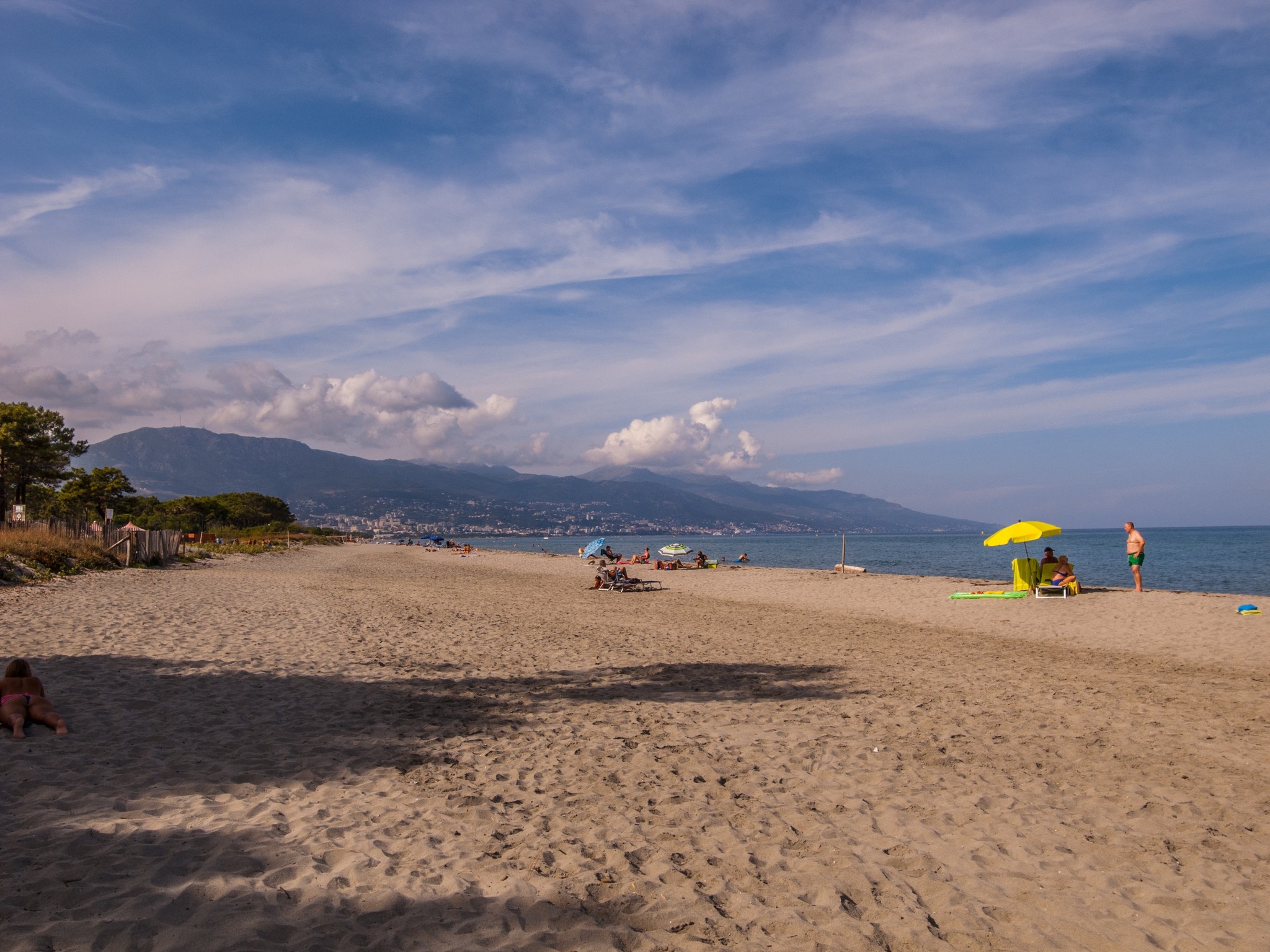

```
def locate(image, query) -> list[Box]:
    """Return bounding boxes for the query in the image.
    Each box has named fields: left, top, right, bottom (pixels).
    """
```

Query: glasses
left=1064, top=558, right=1068, bottom=562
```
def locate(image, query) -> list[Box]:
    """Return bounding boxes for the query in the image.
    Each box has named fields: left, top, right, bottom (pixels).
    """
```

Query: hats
left=595, top=576, right=601, bottom=585
left=588, top=560, right=595, bottom=565
left=1044, top=547, right=1055, bottom=552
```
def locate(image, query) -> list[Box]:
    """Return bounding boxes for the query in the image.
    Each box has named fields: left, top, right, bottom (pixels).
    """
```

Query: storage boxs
left=704, top=560, right=719, bottom=569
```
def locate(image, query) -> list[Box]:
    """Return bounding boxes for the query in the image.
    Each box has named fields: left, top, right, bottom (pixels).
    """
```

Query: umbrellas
left=984, top=519, right=1061, bottom=590
left=115, top=520, right=147, bottom=531
left=582, top=537, right=606, bottom=560
left=658, top=543, right=694, bottom=560
left=89, top=521, right=104, bottom=531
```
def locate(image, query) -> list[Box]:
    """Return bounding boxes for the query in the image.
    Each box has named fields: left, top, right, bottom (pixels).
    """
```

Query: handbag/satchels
left=1069, top=580, right=1081, bottom=596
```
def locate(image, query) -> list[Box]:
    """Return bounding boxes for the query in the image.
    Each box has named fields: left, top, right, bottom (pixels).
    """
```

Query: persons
left=1040, top=547, right=1059, bottom=571
left=657, top=559, right=693, bottom=569
left=0, top=659, right=69, bottom=738
left=606, top=546, right=622, bottom=563
left=542, top=549, right=546, bottom=553
left=357, top=535, right=497, bottom=554
left=630, top=547, right=649, bottom=564
left=1124, top=522, right=1145, bottom=592
left=599, top=560, right=632, bottom=580
left=232, top=537, right=273, bottom=547
left=721, top=557, right=725, bottom=561
left=582, top=546, right=600, bottom=558
left=1050, top=555, right=1076, bottom=586
left=532, top=544, right=534, bottom=546
left=736, top=553, right=750, bottom=562
left=593, top=575, right=638, bottom=588
left=538, top=544, right=540, bottom=546
left=514, top=544, right=517, bottom=547
left=693, top=551, right=708, bottom=568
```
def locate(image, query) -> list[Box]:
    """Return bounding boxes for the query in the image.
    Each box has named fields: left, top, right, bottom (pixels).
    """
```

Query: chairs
left=601, top=548, right=620, bottom=565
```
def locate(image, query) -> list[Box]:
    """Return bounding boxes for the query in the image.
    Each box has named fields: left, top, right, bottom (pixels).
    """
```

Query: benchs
left=432, top=544, right=447, bottom=548
left=1035, top=563, right=1074, bottom=599
left=446, top=541, right=462, bottom=548
left=603, top=578, right=663, bottom=594
left=595, top=567, right=645, bottom=592
